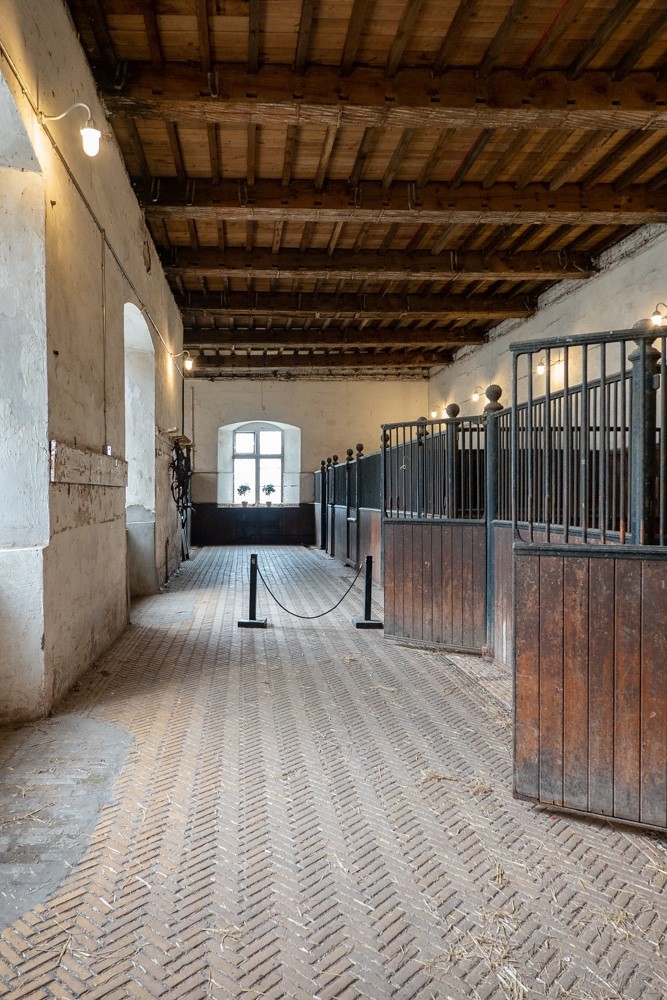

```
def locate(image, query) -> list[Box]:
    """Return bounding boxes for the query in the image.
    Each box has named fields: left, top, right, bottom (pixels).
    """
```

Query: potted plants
left=237, top=485, right=251, bottom=507
left=262, top=483, right=275, bottom=506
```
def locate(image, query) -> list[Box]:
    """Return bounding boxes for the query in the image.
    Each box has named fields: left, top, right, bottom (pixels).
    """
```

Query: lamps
left=651, top=302, right=667, bottom=325
left=472, top=386, right=484, bottom=401
left=171, top=350, right=193, bottom=370
left=41, top=103, right=101, bottom=156
left=431, top=406, right=439, bottom=418
left=537, top=356, right=546, bottom=375
